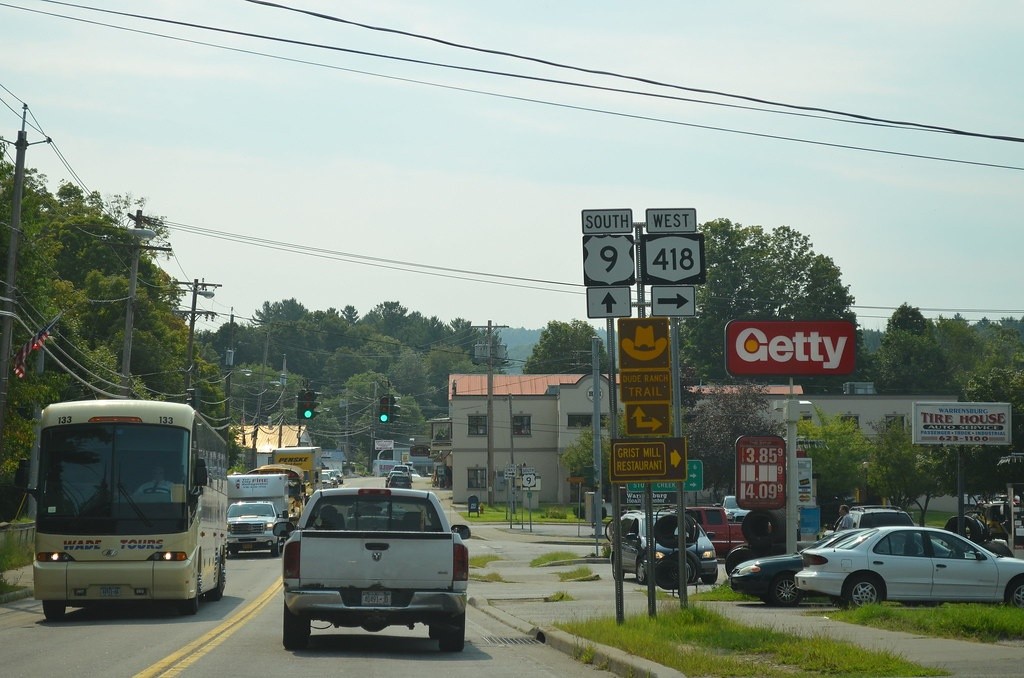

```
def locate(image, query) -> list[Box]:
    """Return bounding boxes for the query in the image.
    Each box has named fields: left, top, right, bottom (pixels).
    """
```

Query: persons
left=838, top=505, right=854, bottom=530
left=132, top=466, right=174, bottom=494
left=1013, top=495, right=1020, bottom=505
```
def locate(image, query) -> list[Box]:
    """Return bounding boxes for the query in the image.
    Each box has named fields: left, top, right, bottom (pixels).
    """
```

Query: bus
left=15, top=399, right=229, bottom=620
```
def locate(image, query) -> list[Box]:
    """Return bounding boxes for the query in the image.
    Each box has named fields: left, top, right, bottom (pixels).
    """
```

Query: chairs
left=403, top=512, right=425, bottom=527
left=322, top=513, right=345, bottom=529
left=904, top=542, right=919, bottom=555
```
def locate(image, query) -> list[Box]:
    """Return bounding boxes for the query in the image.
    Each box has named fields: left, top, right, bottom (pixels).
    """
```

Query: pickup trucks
left=273, top=487, right=474, bottom=654
left=684, top=506, right=748, bottom=556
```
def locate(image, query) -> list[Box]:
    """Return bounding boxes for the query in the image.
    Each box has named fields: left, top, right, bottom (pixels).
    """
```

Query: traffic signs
left=610, top=438, right=689, bottom=484
left=626, top=460, right=705, bottom=493
left=507, top=465, right=517, bottom=478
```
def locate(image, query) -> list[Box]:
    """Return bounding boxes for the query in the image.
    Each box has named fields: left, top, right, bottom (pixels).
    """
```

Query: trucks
left=223, top=448, right=323, bottom=559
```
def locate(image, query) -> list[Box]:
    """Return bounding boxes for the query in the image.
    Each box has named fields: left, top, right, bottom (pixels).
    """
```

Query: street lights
left=0, top=223, right=158, bottom=423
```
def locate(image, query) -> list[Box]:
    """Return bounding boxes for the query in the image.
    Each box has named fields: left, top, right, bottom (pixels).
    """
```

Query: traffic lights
left=297, top=389, right=322, bottom=420
left=379, top=397, right=389, bottom=423
left=390, top=395, right=401, bottom=422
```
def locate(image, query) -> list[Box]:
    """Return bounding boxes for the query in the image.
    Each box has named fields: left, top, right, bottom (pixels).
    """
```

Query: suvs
left=849, top=505, right=915, bottom=529
left=610, top=510, right=720, bottom=585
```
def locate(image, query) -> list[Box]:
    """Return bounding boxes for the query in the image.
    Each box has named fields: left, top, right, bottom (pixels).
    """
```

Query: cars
left=384, top=465, right=420, bottom=490
left=321, top=468, right=343, bottom=489
left=794, top=527, right=1024, bottom=610
left=722, top=495, right=751, bottom=524
left=728, top=528, right=951, bottom=609
